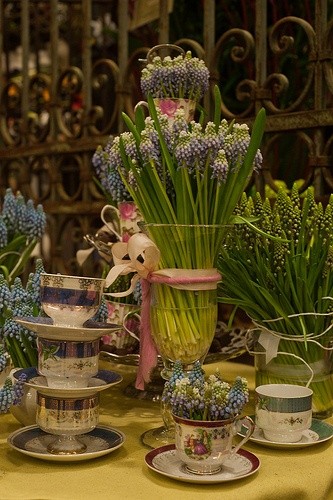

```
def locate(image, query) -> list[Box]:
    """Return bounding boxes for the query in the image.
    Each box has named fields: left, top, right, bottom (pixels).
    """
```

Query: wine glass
left=35, top=389, right=100, bottom=454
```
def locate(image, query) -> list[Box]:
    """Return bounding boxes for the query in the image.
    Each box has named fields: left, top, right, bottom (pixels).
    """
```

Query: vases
left=144, top=303, right=218, bottom=450
left=171, top=412, right=255, bottom=475
left=253, top=341, right=333, bottom=418
left=8, top=367, right=41, bottom=426
left=103, top=301, right=139, bottom=352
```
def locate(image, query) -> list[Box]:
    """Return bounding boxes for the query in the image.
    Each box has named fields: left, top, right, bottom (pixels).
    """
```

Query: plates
left=7, top=424, right=126, bottom=462
left=13, top=317, right=123, bottom=339
left=237, top=415, right=333, bottom=450
left=12, top=367, right=123, bottom=396
left=144, top=444, right=260, bottom=484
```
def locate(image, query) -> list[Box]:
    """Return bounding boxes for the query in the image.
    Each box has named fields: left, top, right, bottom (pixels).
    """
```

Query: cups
left=36, top=337, right=101, bottom=389
left=38, top=273, right=106, bottom=328
left=254, top=384, right=313, bottom=442
left=170, top=410, right=255, bottom=475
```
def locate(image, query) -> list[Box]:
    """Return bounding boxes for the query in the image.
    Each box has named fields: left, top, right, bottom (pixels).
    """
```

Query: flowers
left=0, top=189, right=55, bottom=415
left=164, top=361, right=249, bottom=421
left=87, top=46, right=333, bottom=338
